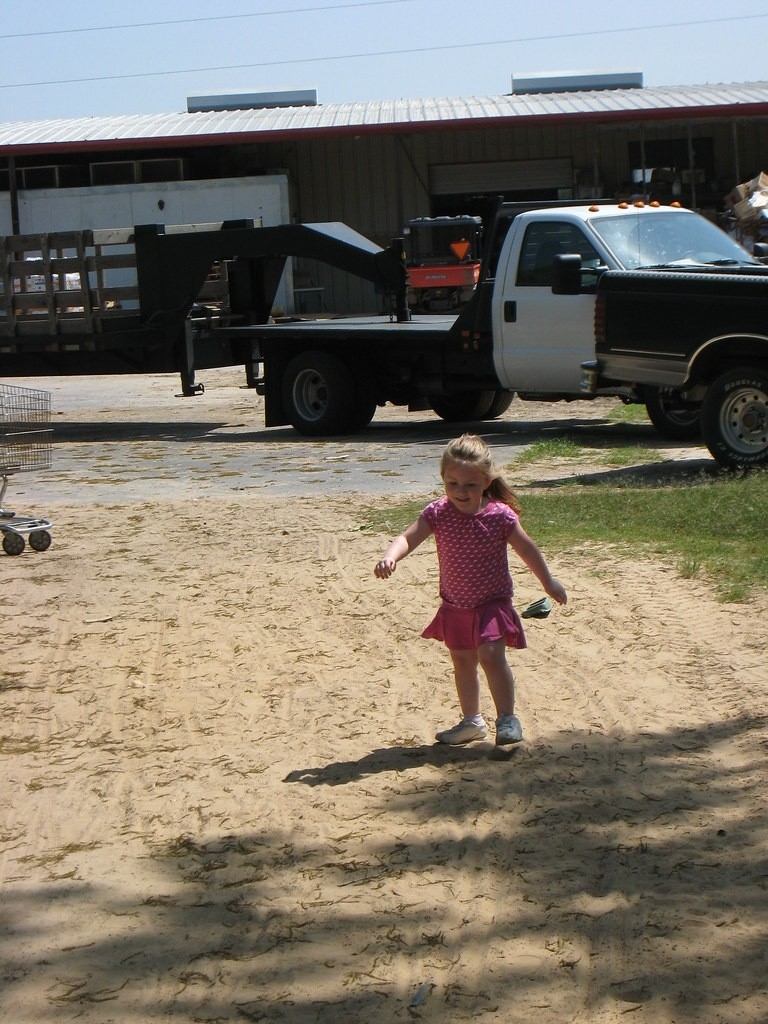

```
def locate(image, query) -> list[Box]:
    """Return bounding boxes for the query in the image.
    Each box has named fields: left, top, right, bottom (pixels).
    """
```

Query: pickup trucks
left=578, top=257, right=766, bottom=475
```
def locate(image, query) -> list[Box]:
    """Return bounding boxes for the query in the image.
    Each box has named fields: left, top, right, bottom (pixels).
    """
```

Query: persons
left=374, top=436, right=568, bottom=744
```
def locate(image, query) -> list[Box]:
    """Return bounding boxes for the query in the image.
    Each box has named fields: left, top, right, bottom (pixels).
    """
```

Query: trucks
left=390, top=216, right=488, bottom=315
left=204, top=198, right=766, bottom=446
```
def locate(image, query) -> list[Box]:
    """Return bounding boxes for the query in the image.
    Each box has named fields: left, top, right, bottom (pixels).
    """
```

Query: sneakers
left=435, top=714, right=488, bottom=745
left=494, top=714, right=522, bottom=745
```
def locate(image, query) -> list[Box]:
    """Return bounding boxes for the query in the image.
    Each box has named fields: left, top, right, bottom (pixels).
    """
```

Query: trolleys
left=0, top=382, right=56, bottom=556
